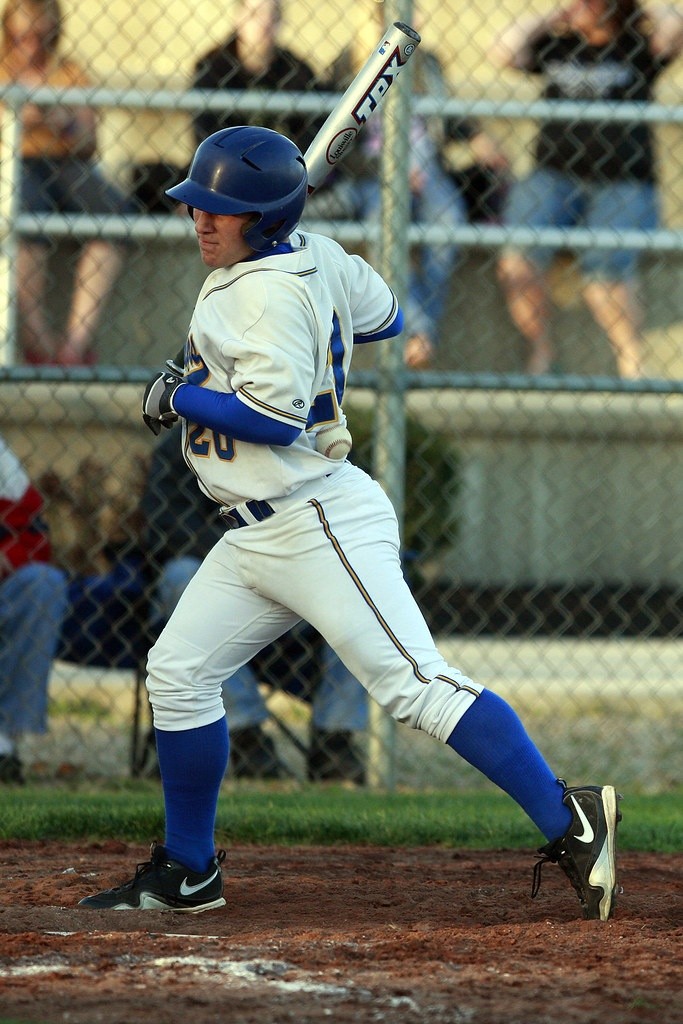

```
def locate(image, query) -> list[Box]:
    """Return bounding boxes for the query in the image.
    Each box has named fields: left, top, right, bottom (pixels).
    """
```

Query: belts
left=217, top=499, right=276, bottom=530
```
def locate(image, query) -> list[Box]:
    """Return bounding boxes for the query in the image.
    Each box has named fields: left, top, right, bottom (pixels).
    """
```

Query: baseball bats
left=164, top=19, right=423, bottom=378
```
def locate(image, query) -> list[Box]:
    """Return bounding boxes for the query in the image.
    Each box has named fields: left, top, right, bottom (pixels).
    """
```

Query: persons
left=500, top=0, right=683, bottom=380
left=191, top=0, right=315, bottom=144
left=331, top=1, right=467, bottom=366
left=0, top=439, right=67, bottom=785
left=73, top=125, right=623, bottom=921
left=0, top=0, right=137, bottom=362
left=143, top=421, right=371, bottom=784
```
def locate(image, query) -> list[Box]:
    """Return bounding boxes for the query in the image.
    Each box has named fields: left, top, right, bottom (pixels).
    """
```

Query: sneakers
left=77, top=839, right=227, bottom=915
left=531, top=777, right=624, bottom=923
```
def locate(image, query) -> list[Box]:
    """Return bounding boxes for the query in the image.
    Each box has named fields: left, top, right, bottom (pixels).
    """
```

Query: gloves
left=141, top=371, right=188, bottom=437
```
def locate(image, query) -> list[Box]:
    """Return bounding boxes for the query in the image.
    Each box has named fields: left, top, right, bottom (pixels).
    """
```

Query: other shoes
left=227, top=723, right=280, bottom=780
left=0, top=754, right=25, bottom=788
left=308, top=730, right=367, bottom=787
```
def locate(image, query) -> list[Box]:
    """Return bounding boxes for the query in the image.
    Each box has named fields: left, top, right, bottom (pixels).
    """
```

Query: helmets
left=165, top=125, right=308, bottom=253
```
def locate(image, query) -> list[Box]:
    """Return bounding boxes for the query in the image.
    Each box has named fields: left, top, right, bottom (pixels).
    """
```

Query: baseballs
left=315, top=423, right=353, bottom=459
